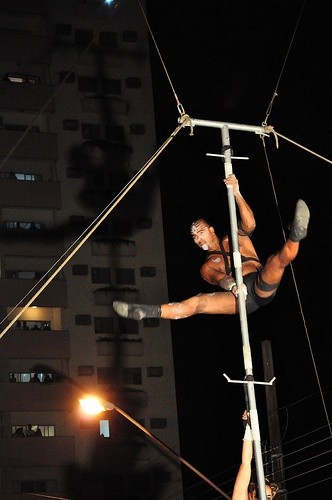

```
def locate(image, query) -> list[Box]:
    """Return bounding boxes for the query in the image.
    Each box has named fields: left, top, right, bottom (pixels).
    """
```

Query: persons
left=12, top=424, right=42, bottom=437
left=14, top=321, right=40, bottom=330
left=9, top=373, right=52, bottom=383
left=112, top=173, right=311, bottom=322
left=231, top=409, right=280, bottom=500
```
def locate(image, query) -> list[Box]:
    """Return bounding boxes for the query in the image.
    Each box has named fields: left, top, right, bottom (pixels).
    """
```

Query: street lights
left=76, top=393, right=231, bottom=500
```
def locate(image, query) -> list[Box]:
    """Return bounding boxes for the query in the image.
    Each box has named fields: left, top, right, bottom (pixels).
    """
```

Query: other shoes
left=289, top=199, right=311, bottom=242
left=113, top=300, right=161, bottom=320
